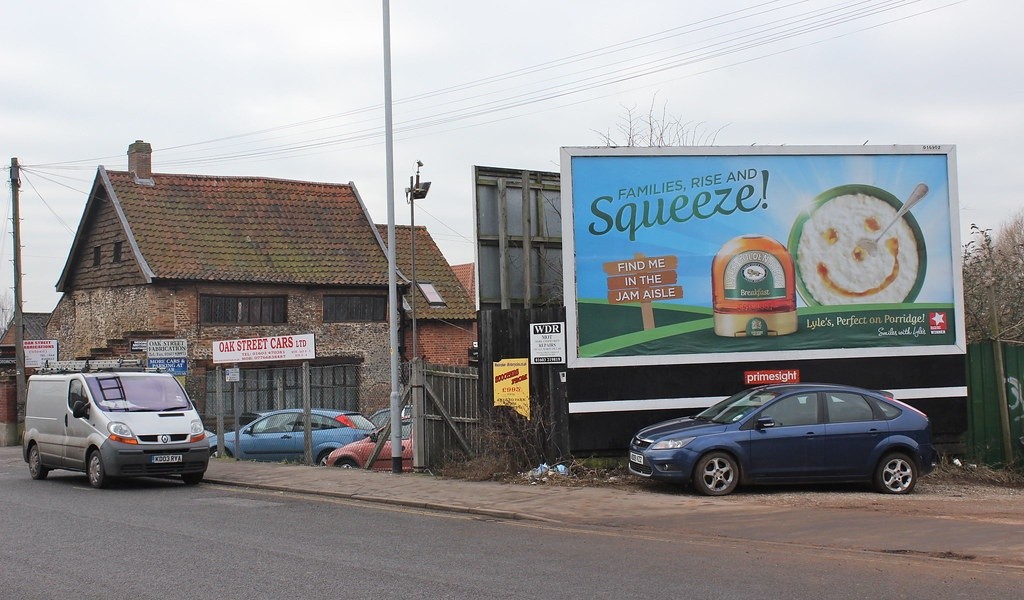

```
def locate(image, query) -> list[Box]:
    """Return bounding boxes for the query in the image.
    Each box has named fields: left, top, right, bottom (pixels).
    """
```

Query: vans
left=22, top=372, right=210, bottom=491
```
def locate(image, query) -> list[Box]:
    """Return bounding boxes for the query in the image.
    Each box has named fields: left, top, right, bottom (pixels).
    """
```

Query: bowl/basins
left=788, top=184, right=927, bottom=308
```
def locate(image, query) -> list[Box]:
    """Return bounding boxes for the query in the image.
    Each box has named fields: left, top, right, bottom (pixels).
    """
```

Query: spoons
left=857, top=184, right=928, bottom=257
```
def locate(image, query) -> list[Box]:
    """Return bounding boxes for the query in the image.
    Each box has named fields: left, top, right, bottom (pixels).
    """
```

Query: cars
left=199, top=411, right=273, bottom=435
left=325, top=415, right=414, bottom=473
left=628, top=383, right=936, bottom=495
left=369, top=406, right=413, bottom=430
left=208, top=409, right=376, bottom=468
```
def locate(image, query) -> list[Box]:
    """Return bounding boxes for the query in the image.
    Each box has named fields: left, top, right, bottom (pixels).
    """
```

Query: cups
left=953, top=459, right=962, bottom=467
